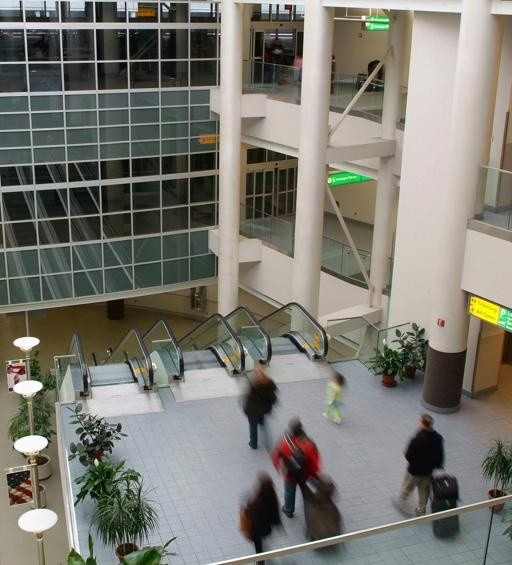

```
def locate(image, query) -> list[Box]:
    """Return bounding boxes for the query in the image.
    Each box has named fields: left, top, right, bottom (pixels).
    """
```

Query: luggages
left=302, top=494, right=346, bottom=550
left=431, top=500, right=459, bottom=537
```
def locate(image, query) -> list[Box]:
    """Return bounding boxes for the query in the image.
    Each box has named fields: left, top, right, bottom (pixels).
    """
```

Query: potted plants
left=480, top=437, right=511, bottom=512
left=364, top=321, right=430, bottom=389
left=9, top=395, right=158, bottom=565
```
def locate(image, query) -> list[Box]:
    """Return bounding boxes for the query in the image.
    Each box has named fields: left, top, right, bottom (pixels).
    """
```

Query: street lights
left=13, top=336, right=58, bottom=565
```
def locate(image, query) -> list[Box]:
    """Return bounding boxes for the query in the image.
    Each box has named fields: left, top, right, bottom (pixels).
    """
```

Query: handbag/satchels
left=431, top=472, right=459, bottom=500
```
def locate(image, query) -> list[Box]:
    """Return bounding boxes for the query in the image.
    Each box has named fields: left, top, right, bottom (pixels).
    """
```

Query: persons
left=271, top=418, right=319, bottom=517
left=393, top=413, right=444, bottom=516
left=321, top=371, right=346, bottom=425
left=245, top=478, right=281, bottom=565
left=244, top=369, right=276, bottom=450
